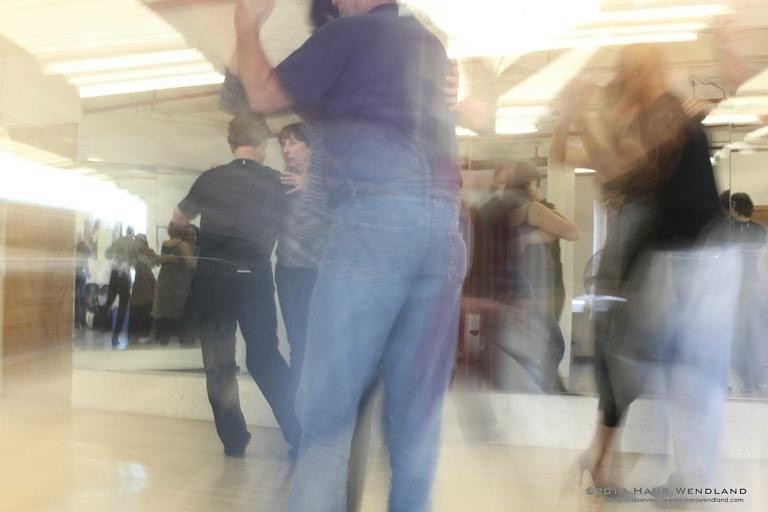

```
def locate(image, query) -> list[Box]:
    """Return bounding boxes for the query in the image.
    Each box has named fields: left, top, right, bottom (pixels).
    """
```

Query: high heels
left=578, top=449, right=637, bottom=501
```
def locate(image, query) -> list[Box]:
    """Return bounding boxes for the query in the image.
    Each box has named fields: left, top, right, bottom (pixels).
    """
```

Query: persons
left=76, top=216, right=199, bottom=349
left=167, top=0, right=768, bottom=511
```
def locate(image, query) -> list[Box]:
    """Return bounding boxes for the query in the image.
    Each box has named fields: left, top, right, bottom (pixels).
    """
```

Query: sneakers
left=288, top=443, right=309, bottom=461
left=223, top=431, right=252, bottom=456
